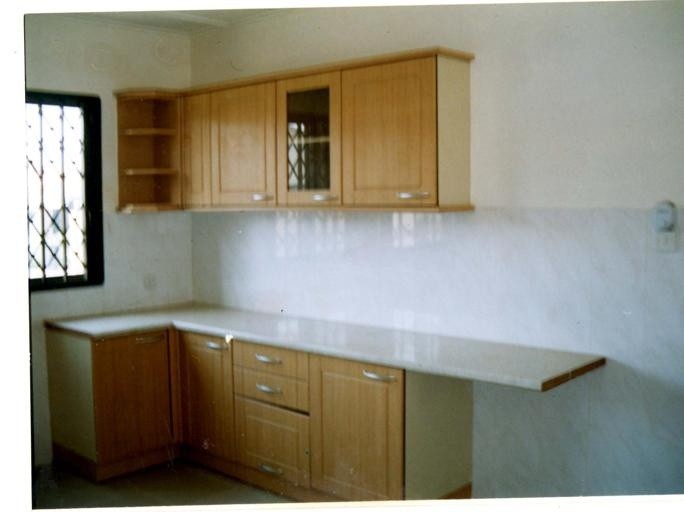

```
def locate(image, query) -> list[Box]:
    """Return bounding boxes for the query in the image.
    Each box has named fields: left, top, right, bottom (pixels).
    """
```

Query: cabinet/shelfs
left=170, top=318, right=237, bottom=485
left=42, top=313, right=177, bottom=487
left=271, top=59, right=342, bottom=211
left=207, top=72, right=276, bottom=214
left=172, top=85, right=212, bottom=219
left=340, top=46, right=475, bottom=216
left=306, top=345, right=475, bottom=502
left=228, top=332, right=312, bottom=502
left=110, top=80, right=185, bottom=218
